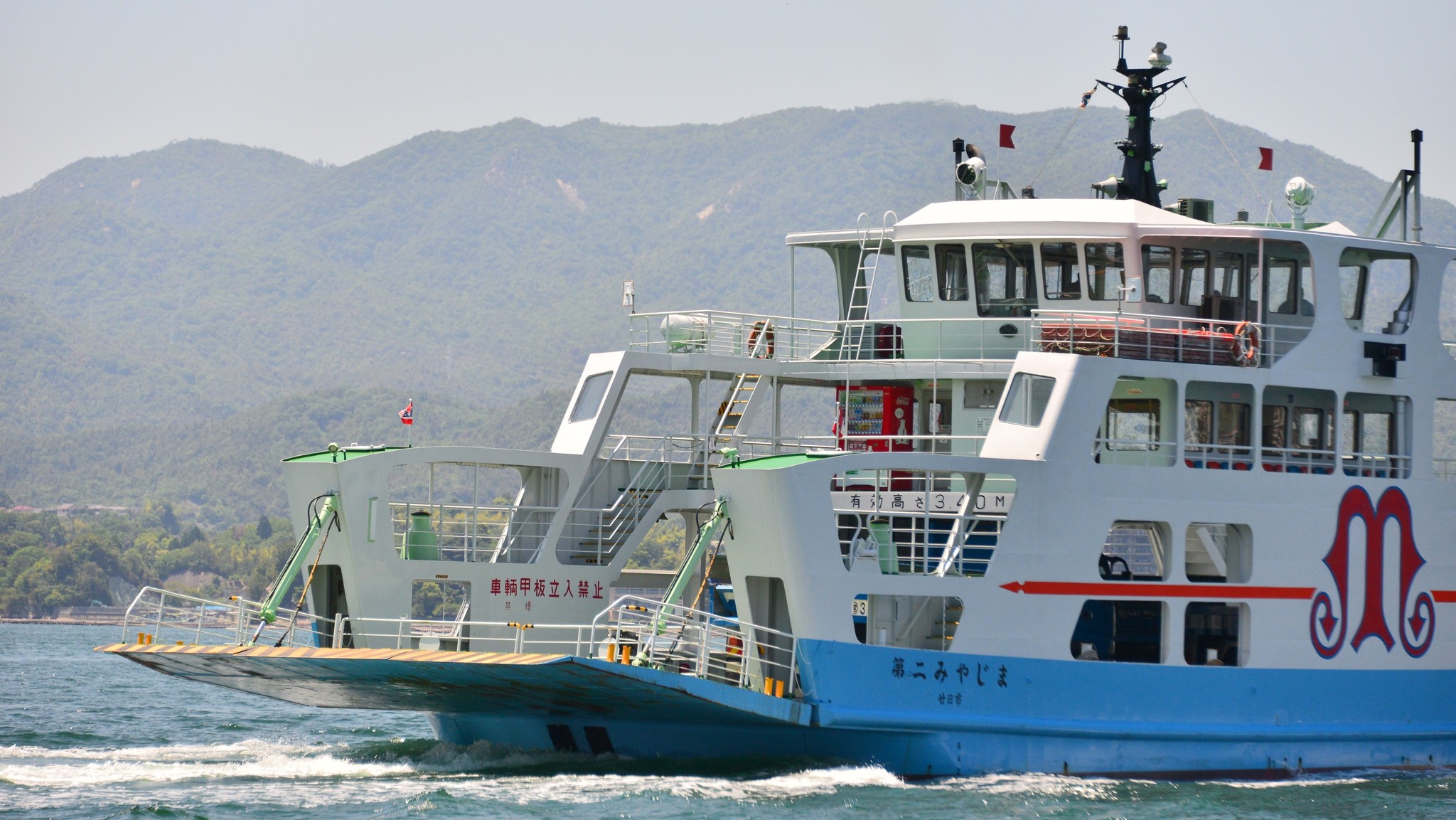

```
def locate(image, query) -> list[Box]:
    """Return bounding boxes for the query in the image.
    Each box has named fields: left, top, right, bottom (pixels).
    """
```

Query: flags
left=397, top=401, right=413, bottom=425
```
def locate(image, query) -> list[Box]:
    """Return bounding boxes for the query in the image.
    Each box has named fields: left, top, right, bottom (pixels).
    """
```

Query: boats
left=86, top=21, right=1456, bottom=789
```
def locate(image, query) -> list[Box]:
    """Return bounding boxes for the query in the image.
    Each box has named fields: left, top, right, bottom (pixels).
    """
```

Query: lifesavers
left=748, top=322, right=774, bottom=359
left=1234, top=321, right=1258, bottom=367
left=879, top=326, right=902, bottom=358
